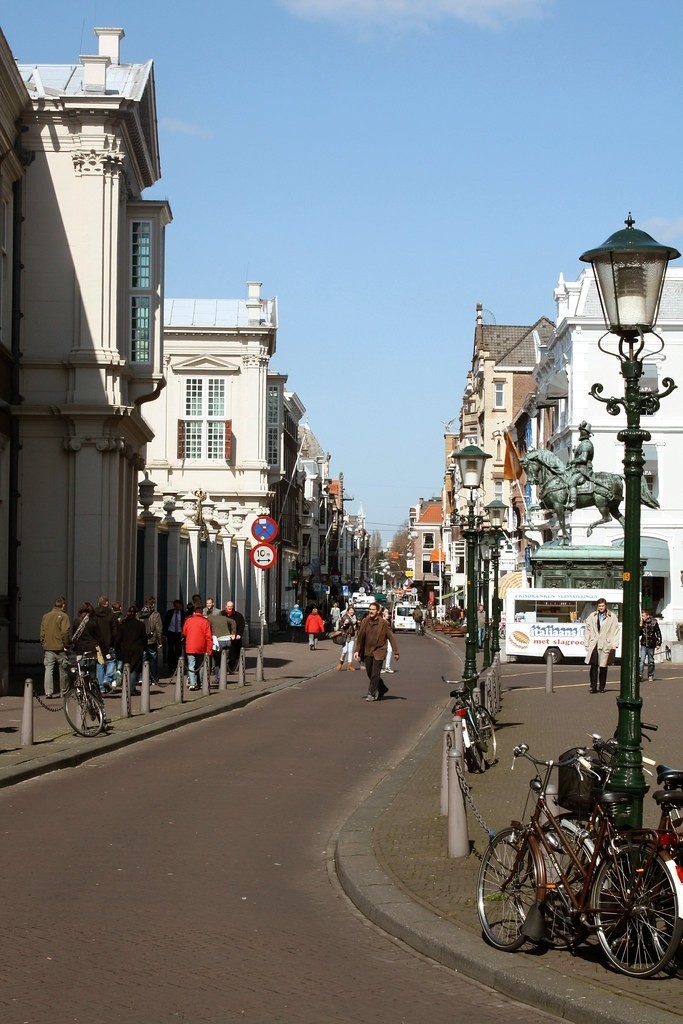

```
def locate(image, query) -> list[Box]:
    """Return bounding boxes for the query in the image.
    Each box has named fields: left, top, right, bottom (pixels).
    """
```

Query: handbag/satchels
left=96, top=646, right=105, bottom=665
left=333, top=625, right=346, bottom=646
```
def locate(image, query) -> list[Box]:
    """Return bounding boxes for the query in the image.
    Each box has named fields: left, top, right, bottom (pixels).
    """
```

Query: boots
left=346, top=661, right=355, bottom=672
left=337, top=659, right=343, bottom=671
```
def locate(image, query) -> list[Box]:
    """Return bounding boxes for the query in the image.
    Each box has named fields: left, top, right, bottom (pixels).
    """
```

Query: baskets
left=557, top=747, right=607, bottom=811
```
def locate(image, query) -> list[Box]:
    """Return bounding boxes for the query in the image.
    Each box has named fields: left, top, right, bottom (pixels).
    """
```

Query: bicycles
left=442, top=668, right=501, bottom=773
left=64, top=648, right=114, bottom=736
left=470, top=747, right=683, bottom=986
left=418, top=622, right=423, bottom=636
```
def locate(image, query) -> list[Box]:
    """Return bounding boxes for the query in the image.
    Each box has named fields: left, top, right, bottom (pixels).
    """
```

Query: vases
left=434, top=625, right=447, bottom=631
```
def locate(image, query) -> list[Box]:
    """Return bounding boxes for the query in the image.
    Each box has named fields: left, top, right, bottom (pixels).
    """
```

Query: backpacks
left=142, top=616, right=155, bottom=637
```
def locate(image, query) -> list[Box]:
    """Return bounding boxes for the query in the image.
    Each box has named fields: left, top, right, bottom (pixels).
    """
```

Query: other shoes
left=366, top=694, right=375, bottom=701
left=385, top=668, right=394, bottom=674
left=599, top=687, right=605, bottom=693
left=648, top=675, right=653, bottom=682
left=640, top=675, right=643, bottom=681
left=46, top=695, right=51, bottom=699
left=377, top=688, right=389, bottom=701
left=590, top=688, right=597, bottom=693
left=380, top=669, right=386, bottom=674
left=103, top=681, right=113, bottom=691
left=188, top=686, right=195, bottom=690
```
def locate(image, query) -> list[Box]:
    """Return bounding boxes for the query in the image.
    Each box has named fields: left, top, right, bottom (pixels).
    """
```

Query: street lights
left=477, top=532, right=495, bottom=674
left=580, top=213, right=679, bottom=843
left=450, top=445, right=494, bottom=699
left=483, top=498, right=510, bottom=676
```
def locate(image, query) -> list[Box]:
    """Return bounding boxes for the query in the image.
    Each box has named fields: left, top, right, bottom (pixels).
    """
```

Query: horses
left=519, top=449, right=661, bottom=547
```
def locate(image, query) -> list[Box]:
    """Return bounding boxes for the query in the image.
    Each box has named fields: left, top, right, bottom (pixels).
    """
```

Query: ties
left=175, top=610, right=178, bottom=635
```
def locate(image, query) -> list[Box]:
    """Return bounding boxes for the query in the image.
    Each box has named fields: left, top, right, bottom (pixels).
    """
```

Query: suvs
left=392, top=606, right=418, bottom=633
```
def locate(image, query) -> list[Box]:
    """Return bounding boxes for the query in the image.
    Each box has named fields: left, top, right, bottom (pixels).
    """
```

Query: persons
left=305, top=607, right=325, bottom=650
left=337, top=605, right=400, bottom=674
left=289, top=604, right=303, bottom=643
left=560, top=422, right=593, bottom=512
left=639, top=609, right=662, bottom=682
left=584, top=598, right=618, bottom=693
left=330, top=601, right=341, bottom=631
left=353, top=602, right=400, bottom=703
left=430, top=604, right=486, bottom=649
left=412, top=605, right=425, bottom=636
left=40, top=593, right=245, bottom=700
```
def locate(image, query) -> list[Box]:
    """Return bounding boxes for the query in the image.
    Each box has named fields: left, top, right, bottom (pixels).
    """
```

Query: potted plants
left=442, top=606, right=465, bottom=637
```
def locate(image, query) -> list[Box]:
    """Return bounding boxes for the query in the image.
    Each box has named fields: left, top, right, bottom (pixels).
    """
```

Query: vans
left=503, top=587, right=672, bottom=668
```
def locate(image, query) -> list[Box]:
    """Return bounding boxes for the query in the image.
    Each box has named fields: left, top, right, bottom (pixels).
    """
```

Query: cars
left=346, top=590, right=390, bottom=629
